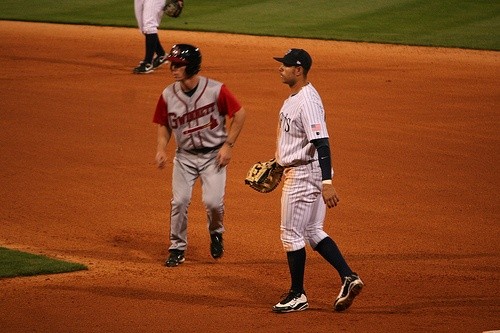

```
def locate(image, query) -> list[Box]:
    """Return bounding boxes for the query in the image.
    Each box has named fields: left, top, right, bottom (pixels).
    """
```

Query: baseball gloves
left=244, top=158, right=284, bottom=193
left=163, top=0, right=185, bottom=18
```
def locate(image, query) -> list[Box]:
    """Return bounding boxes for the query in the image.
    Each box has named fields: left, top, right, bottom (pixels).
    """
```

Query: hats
left=273, top=48, right=313, bottom=70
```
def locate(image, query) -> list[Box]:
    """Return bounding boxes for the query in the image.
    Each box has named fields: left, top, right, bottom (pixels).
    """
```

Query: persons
left=132, top=0, right=184, bottom=74
left=246, top=49, right=364, bottom=313
left=153, top=44, right=246, bottom=267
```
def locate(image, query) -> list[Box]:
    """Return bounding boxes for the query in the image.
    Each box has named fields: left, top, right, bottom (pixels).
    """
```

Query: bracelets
left=225, top=140, right=234, bottom=148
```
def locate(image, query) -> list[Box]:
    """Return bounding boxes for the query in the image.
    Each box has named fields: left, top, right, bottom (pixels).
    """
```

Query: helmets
left=160, top=44, right=202, bottom=77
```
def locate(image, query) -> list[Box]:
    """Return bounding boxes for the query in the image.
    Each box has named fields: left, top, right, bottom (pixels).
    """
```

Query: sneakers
left=153, top=52, right=170, bottom=69
left=134, top=61, right=154, bottom=73
left=272, top=289, right=310, bottom=312
left=210, top=232, right=223, bottom=258
left=334, top=272, right=364, bottom=311
left=165, top=250, right=185, bottom=266
